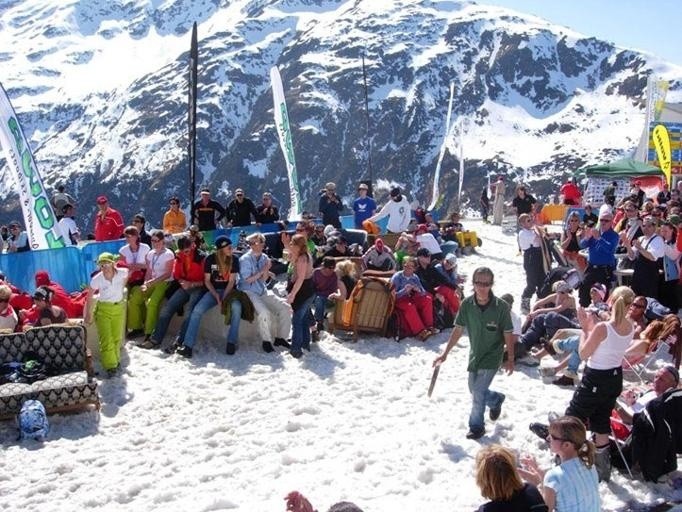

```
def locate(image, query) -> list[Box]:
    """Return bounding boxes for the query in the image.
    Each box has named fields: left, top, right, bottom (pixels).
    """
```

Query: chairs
left=622, top=335, right=674, bottom=385
left=328, top=276, right=396, bottom=343
left=589, top=389, right=682, bottom=480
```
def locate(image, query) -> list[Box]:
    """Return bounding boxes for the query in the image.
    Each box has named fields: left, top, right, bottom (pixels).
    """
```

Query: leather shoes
left=263, top=338, right=290, bottom=353
left=123, top=329, right=194, bottom=358
left=226, top=342, right=235, bottom=354
left=466, top=425, right=485, bottom=439
left=489, top=393, right=505, bottom=420
left=529, top=421, right=549, bottom=439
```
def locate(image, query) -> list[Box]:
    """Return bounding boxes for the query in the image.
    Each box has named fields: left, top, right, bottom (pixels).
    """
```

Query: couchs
left=234, top=229, right=368, bottom=274
left=0, top=324, right=104, bottom=424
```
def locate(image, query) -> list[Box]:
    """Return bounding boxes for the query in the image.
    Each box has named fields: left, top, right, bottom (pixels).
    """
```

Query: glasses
left=475, top=281, right=491, bottom=287
left=169, top=202, right=175, bottom=205
left=551, top=435, right=566, bottom=441
left=0, top=298, right=9, bottom=303
left=600, top=219, right=611, bottom=223
left=632, top=303, right=645, bottom=309
left=150, top=239, right=160, bottom=243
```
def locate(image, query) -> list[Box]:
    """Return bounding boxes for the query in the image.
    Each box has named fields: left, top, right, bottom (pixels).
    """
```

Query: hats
left=201, top=189, right=244, bottom=195
left=376, top=238, right=384, bottom=253
left=215, top=234, right=232, bottom=249
left=445, top=252, right=457, bottom=267
left=98, top=252, right=114, bottom=264
left=0, top=285, right=12, bottom=299
left=591, top=282, right=607, bottom=300
left=34, top=272, right=50, bottom=301
left=96, top=195, right=108, bottom=205
left=642, top=197, right=682, bottom=224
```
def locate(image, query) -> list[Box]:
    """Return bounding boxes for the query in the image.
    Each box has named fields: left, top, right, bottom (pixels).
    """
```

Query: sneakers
left=518, top=337, right=574, bottom=387
left=419, top=330, right=433, bottom=342
left=312, top=331, right=320, bottom=342
left=521, top=297, right=530, bottom=315
